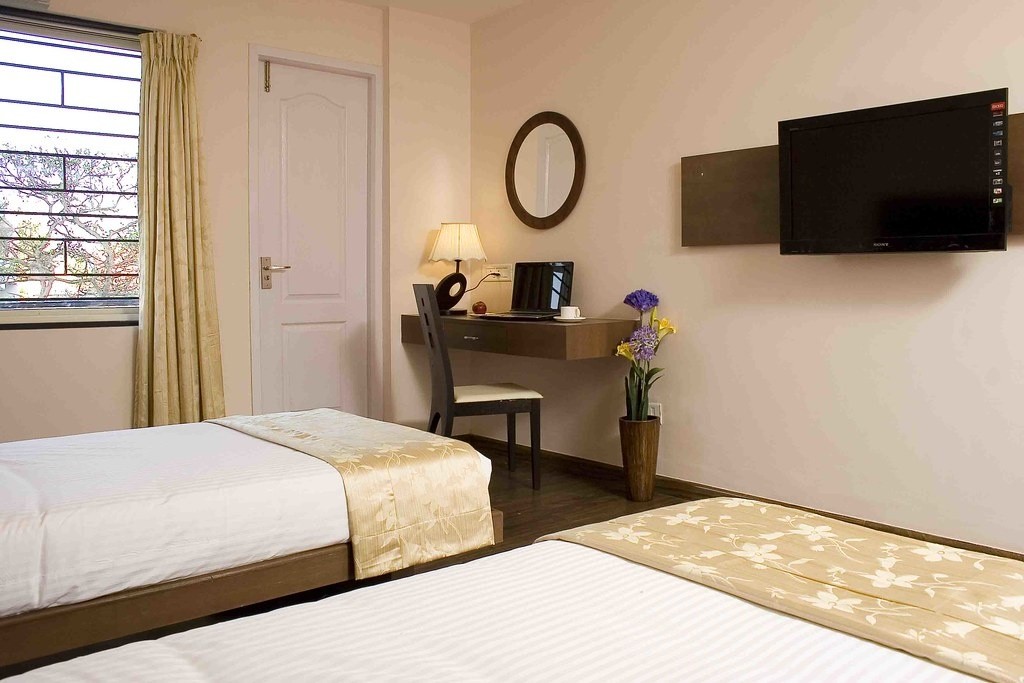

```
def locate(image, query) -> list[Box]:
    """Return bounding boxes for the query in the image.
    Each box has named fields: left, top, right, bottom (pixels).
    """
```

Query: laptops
left=471, top=261, right=575, bottom=320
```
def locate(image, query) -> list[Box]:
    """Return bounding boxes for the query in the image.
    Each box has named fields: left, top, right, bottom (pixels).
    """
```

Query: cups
left=561, top=307, right=580, bottom=318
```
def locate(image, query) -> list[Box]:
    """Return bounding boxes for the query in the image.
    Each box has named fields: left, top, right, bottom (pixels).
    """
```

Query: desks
left=401, top=312, right=640, bottom=361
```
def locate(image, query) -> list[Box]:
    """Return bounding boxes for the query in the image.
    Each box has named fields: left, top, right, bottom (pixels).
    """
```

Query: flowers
left=615, top=289, right=677, bottom=421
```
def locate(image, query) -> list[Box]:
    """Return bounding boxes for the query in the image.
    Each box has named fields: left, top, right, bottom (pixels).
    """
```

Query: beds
left=0, top=408, right=503, bottom=667
left=0, top=497, right=1024, bottom=682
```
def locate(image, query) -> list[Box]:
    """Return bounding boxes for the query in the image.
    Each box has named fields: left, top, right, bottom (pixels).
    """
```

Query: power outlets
left=648, top=403, right=662, bottom=425
left=482, top=264, right=513, bottom=282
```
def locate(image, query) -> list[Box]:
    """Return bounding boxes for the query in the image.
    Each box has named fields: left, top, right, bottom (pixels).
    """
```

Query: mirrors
left=505, top=110, right=587, bottom=230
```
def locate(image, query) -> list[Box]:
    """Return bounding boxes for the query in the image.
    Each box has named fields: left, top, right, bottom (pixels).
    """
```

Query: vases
left=618, top=415, right=660, bottom=502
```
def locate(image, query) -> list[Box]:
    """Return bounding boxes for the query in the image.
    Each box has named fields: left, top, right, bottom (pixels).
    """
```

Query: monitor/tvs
left=778, top=87, right=1008, bottom=255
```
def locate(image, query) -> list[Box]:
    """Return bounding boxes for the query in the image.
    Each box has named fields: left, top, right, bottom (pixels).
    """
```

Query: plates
left=554, top=317, right=586, bottom=322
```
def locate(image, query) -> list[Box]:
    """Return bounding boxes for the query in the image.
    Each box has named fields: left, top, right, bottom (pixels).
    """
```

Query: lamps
left=428, top=223, right=501, bottom=315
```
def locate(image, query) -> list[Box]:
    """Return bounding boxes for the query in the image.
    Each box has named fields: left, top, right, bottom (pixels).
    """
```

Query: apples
left=472, top=301, right=486, bottom=314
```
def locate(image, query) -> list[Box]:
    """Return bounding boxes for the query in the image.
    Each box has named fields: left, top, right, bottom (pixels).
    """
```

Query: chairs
left=413, top=284, right=544, bottom=490
